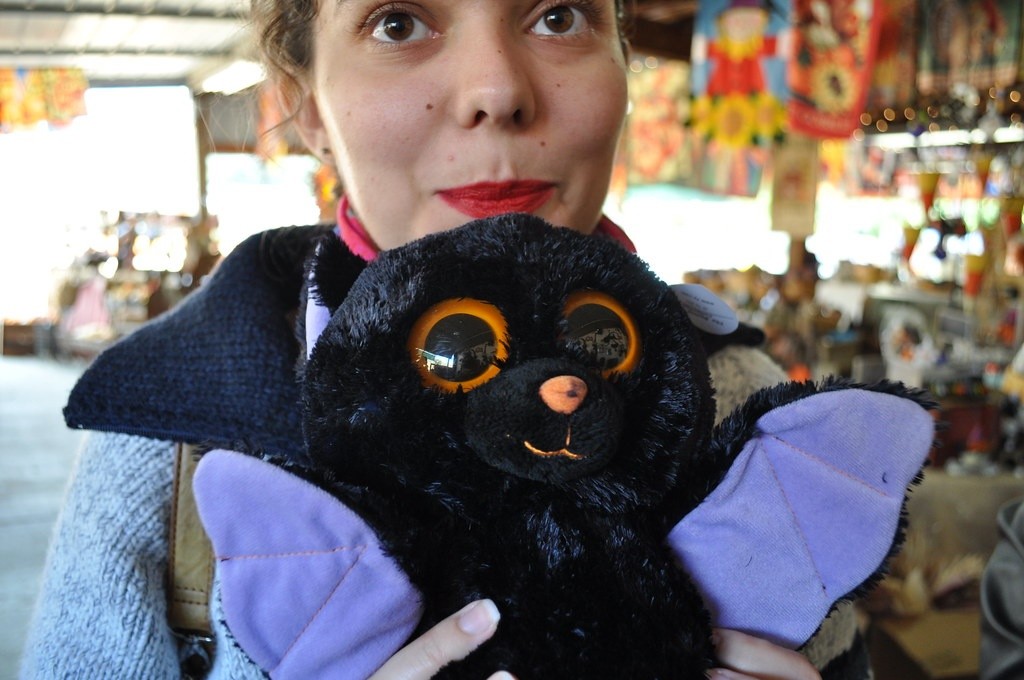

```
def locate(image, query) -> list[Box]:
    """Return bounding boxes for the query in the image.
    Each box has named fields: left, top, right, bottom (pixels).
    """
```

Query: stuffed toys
left=192, top=214, right=938, bottom=680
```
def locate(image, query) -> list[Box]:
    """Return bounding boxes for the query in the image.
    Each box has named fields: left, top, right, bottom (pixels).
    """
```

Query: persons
left=18, top=0, right=874, bottom=680
